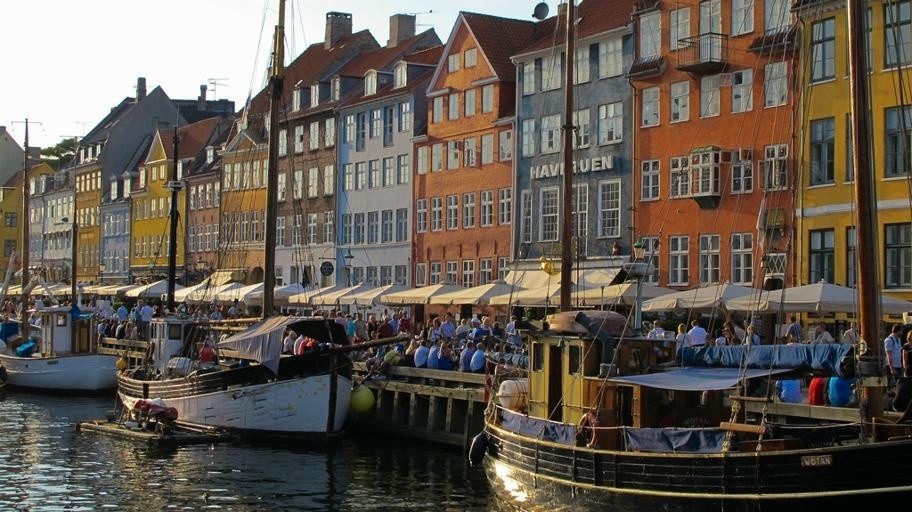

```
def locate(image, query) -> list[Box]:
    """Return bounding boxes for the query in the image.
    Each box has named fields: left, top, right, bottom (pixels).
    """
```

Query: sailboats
left=0, top=119, right=126, bottom=399
left=112, top=0, right=409, bottom=441
left=463, top=1, right=912, bottom=511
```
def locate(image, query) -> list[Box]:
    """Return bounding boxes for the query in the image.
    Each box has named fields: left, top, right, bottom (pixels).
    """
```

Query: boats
left=73, top=415, right=230, bottom=446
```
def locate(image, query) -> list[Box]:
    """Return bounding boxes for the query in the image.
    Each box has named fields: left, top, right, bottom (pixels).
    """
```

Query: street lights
left=342, top=244, right=355, bottom=315
left=631, top=234, right=648, bottom=263
left=98, top=256, right=108, bottom=285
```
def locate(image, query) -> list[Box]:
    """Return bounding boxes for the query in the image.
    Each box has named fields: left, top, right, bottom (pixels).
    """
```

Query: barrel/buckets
left=498, top=379, right=529, bottom=410
left=166, top=357, right=197, bottom=378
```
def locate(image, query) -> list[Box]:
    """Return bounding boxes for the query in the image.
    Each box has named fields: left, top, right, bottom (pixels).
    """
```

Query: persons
left=1, top=297, right=70, bottom=326
left=81, top=296, right=251, bottom=361
left=647, top=320, right=912, bottom=414
left=274, top=310, right=514, bottom=373
